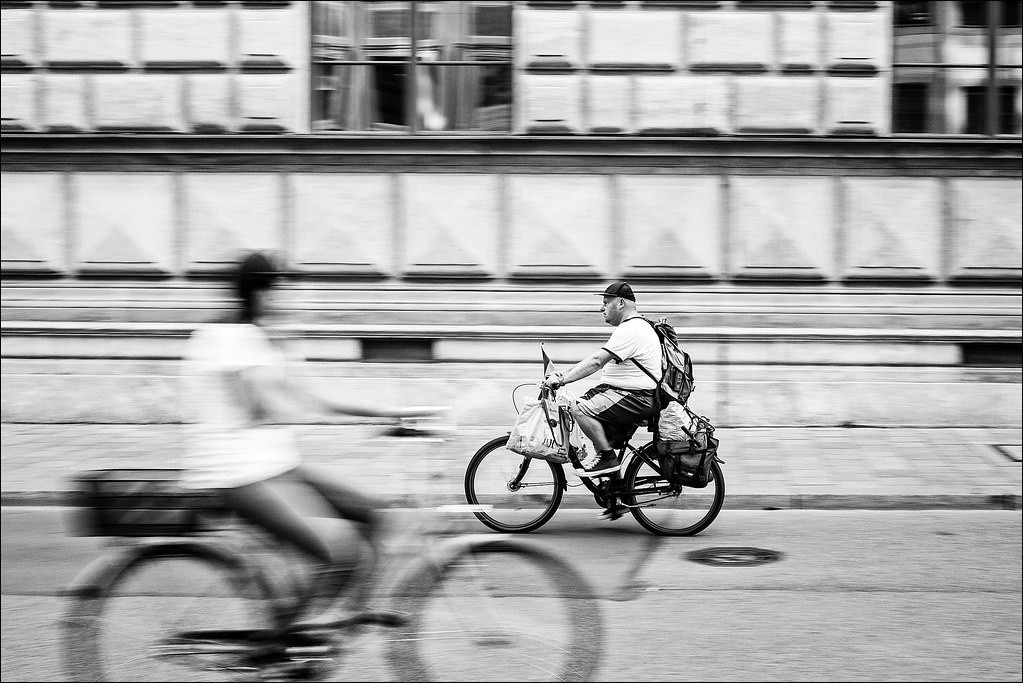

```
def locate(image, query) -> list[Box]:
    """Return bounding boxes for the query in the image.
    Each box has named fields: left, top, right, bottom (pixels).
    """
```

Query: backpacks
left=620, top=316, right=697, bottom=407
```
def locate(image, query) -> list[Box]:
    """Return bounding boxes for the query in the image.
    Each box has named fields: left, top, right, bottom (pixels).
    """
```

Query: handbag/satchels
left=656, top=428, right=719, bottom=488
left=506, top=386, right=574, bottom=464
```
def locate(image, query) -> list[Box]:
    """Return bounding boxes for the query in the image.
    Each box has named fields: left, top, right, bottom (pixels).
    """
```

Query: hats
left=234, top=252, right=288, bottom=294
left=593, top=282, right=635, bottom=302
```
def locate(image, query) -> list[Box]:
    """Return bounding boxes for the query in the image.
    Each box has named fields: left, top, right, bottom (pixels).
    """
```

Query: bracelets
left=556, top=375, right=564, bottom=384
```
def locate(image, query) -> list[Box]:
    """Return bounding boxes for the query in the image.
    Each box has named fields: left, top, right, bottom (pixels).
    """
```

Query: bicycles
left=464, top=355, right=724, bottom=537
left=63, top=410, right=603, bottom=683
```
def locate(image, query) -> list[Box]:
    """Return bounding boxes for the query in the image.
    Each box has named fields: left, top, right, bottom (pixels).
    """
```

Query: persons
left=540, top=281, right=664, bottom=522
left=175, top=251, right=400, bottom=683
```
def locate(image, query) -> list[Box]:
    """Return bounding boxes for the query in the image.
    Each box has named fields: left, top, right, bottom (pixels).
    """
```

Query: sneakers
left=597, top=505, right=630, bottom=519
left=574, top=453, right=621, bottom=477
